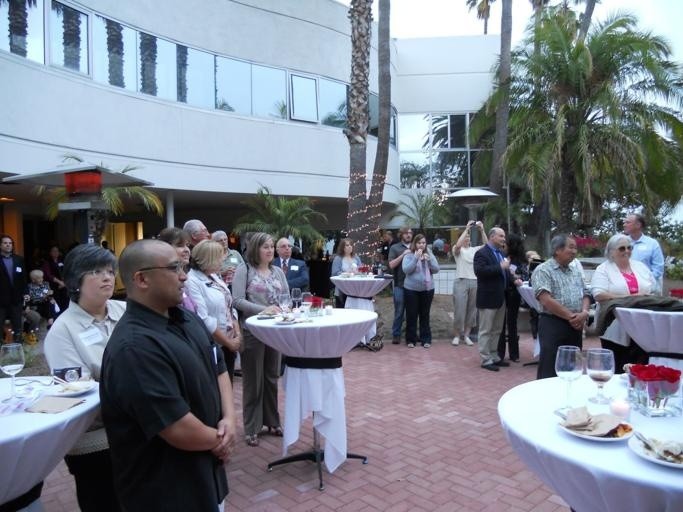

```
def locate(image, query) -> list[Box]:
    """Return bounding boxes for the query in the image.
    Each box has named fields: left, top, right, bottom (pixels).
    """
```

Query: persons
left=0, top=220, right=310, bottom=512
left=452, top=213, right=665, bottom=378
left=325, top=225, right=451, bottom=349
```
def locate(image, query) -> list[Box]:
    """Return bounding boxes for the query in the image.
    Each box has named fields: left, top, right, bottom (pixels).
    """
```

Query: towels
left=24, top=395, right=85, bottom=414
left=566, top=403, right=627, bottom=431
left=634, top=428, right=683, bottom=464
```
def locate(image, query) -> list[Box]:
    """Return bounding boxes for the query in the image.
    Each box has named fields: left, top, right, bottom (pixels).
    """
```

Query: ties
left=495, top=248, right=502, bottom=263
left=282, top=259, right=288, bottom=276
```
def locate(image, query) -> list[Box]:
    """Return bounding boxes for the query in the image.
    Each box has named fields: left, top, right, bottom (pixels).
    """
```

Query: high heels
left=267, top=425, right=282, bottom=436
left=244, top=433, right=257, bottom=446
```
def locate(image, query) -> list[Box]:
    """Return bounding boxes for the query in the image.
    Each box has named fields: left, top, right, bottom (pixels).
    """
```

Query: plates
left=40, top=380, right=99, bottom=397
left=556, top=415, right=682, bottom=472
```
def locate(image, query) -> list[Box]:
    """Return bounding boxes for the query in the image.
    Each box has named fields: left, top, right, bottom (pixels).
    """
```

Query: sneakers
left=14, top=330, right=22, bottom=342
left=391, top=334, right=430, bottom=348
left=451, top=336, right=459, bottom=345
left=463, top=336, right=473, bottom=346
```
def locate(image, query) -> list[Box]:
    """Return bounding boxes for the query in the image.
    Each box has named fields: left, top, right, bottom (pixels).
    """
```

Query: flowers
left=358, top=266, right=368, bottom=274
left=303, top=295, right=322, bottom=313
left=622, top=363, right=681, bottom=410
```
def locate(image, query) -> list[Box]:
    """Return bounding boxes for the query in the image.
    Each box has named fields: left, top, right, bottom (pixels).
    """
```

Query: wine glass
left=0, top=340, right=26, bottom=406
left=274, top=288, right=327, bottom=325
left=553, top=345, right=615, bottom=405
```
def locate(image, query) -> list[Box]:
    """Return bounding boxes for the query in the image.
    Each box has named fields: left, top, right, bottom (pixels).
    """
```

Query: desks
left=245, top=308, right=378, bottom=490
left=0, top=374, right=100, bottom=511
left=496, top=308, right=683, bottom=512
left=329, top=274, right=392, bottom=351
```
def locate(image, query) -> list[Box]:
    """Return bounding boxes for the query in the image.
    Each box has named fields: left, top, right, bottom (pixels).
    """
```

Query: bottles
left=372, top=254, right=384, bottom=276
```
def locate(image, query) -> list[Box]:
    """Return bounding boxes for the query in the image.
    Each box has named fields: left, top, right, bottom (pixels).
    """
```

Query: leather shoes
left=480, top=353, right=520, bottom=370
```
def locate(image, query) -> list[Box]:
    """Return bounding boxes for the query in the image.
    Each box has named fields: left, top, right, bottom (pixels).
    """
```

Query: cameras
left=470, top=221, right=477, bottom=226
left=53, top=366, right=81, bottom=384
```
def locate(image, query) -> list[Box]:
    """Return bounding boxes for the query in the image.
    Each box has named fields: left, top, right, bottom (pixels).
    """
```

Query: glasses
left=73, top=268, right=118, bottom=277
left=131, top=262, right=187, bottom=281
left=614, top=246, right=633, bottom=252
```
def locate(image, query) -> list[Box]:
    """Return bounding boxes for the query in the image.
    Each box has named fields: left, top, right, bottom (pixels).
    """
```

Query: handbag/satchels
left=226, top=283, right=247, bottom=322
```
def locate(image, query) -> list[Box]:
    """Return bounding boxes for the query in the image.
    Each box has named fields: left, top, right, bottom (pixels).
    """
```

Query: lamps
left=64, top=169, right=102, bottom=197
